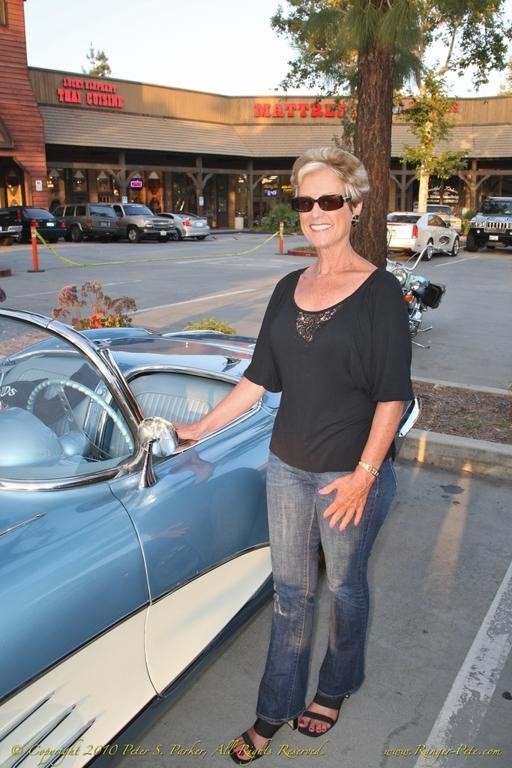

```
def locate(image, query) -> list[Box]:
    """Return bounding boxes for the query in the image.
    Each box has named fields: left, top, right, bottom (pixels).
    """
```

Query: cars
left=387, top=208, right=460, bottom=261
left=154, top=210, right=210, bottom=242
left=0, top=205, right=68, bottom=244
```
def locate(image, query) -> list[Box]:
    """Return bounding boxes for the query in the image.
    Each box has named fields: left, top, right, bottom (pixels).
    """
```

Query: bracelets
left=358, top=460, right=380, bottom=479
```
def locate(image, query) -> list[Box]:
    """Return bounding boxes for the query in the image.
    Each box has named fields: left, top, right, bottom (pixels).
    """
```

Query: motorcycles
left=384, top=226, right=452, bottom=351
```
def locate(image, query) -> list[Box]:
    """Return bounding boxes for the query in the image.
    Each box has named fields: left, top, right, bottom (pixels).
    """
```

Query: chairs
left=0, top=357, right=102, bottom=440
left=99, top=373, right=243, bottom=471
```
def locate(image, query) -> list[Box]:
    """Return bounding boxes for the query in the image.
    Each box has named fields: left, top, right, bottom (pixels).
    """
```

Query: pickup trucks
left=0, top=206, right=23, bottom=244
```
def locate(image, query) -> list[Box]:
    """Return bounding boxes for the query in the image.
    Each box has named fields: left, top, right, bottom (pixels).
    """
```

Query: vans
left=97, top=202, right=176, bottom=243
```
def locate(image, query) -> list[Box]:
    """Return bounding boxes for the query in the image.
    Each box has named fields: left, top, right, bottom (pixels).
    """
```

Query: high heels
left=228, top=715, right=298, bottom=764
left=299, top=693, right=341, bottom=737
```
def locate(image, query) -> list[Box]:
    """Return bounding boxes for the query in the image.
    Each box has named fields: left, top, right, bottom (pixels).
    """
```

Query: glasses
left=291, top=195, right=350, bottom=212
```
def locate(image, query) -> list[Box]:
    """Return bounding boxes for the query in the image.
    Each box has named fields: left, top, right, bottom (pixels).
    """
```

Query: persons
left=166, top=144, right=415, bottom=766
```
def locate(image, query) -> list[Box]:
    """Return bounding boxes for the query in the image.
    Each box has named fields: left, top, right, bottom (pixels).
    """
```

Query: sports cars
left=1, top=304, right=420, bottom=767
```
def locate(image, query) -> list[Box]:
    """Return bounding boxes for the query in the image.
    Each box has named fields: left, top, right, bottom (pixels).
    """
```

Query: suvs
left=466, top=196, right=512, bottom=253
left=50, top=203, right=120, bottom=242
left=414, top=202, right=462, bottom=232
